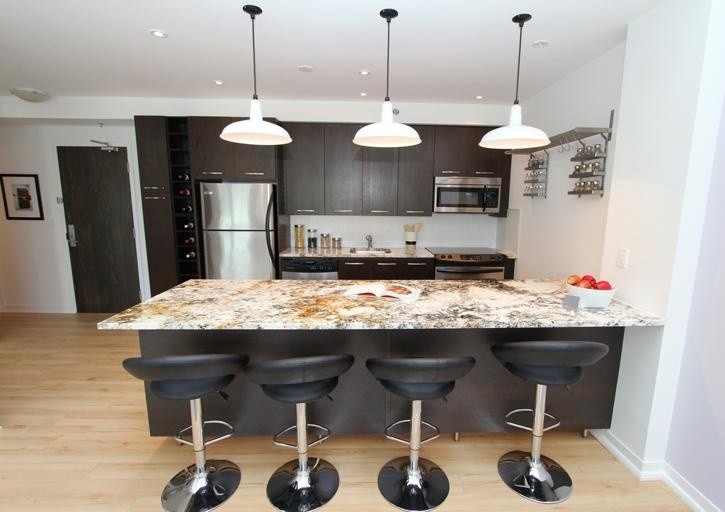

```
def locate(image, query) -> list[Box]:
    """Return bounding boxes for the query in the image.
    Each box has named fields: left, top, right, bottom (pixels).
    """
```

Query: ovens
left=427, top=247, right=515, bottom=280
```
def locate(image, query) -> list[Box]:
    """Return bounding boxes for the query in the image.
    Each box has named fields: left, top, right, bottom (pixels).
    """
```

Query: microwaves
left=433, top=174, right=501, bottom=214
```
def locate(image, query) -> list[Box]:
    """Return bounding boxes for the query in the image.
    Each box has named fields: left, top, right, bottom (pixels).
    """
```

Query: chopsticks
left=404, top=223, right=422, bottom=233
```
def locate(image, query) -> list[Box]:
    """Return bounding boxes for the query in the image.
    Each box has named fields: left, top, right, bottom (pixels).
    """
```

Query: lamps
left=478, top=14, right=551, bottom=149
left=352, top=9, right=421, bottom=148
left=218, top=5, right=292, bottom=145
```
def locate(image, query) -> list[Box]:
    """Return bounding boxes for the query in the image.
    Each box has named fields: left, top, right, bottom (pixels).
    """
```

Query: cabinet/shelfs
left=434, top=125, right=503, bottom=176
left=504, top=109, right=615, bottom=198
left=324, top=123, right=362, bottom=215
left=133, top=116, right=205, bottom=296
left=337, top=258, right=435, bottom=279
left=188, top=116, right=281, bottom=180
left=397, top=124, right=435, bottom=216
left=282, top=122, right=325, bottom=215
left=362, top=123, right=398, bottom=216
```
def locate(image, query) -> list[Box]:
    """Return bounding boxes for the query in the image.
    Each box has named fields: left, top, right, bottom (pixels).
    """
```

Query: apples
left=569, top=275, right=613, bottom=290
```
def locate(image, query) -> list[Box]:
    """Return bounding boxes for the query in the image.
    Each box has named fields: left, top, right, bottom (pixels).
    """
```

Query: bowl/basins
left=566, top=280, right=616, bottom=308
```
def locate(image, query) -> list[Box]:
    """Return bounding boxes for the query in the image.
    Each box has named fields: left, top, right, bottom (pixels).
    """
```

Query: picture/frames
left=0, top=174, right=44, bottom=220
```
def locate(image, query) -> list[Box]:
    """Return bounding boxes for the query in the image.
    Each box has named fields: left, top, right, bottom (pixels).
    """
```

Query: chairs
left=364, top=356, right=476, bottom=511
left=246, top=353, right=354, bottom=511
left=490, top=340, right=609, bottom=503
left=122, top=353, right=250, bottom=511
